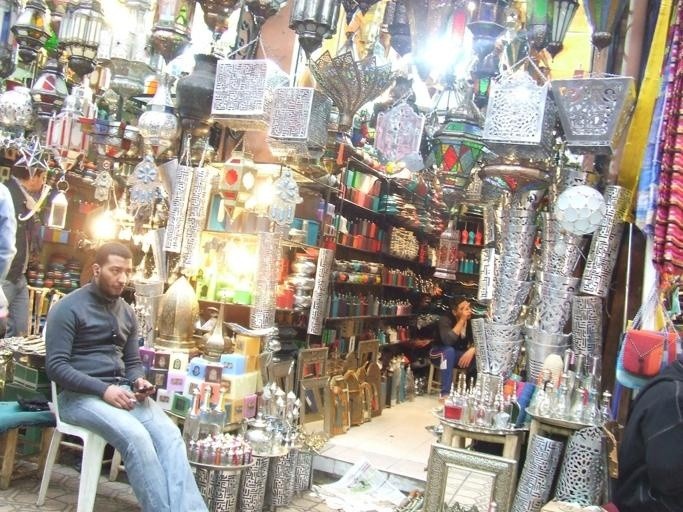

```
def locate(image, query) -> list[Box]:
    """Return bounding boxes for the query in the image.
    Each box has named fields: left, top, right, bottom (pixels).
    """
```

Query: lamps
left=0, top=0, right=639, bottom=238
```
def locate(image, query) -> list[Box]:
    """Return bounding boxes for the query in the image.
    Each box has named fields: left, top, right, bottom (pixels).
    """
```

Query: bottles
left=206, top=193, right=386, bottom=253
left=458, top=221, right=482, bottom=274
left=323, top=353, right=425, bottom=436
left=330, top=267, right=434, bottom=345
left=443, top=348, right=613, bottom=431
left=181, top=385, right=294, bottom=464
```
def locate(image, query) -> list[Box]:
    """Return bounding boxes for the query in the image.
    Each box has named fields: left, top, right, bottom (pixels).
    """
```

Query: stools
left=426, top=362, right=466, bottom=396
left=0, top=400, right=56, bottom=491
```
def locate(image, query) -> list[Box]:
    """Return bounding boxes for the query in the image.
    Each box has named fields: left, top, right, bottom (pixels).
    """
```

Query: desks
left=439, top=404, right=593, bottom=464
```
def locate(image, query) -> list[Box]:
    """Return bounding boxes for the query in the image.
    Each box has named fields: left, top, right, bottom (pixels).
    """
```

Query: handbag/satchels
left=616, top=288, right=681, bottom=390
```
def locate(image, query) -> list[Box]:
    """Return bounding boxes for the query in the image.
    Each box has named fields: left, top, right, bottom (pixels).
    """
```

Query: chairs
left=35, top=380, right=127, bottom=511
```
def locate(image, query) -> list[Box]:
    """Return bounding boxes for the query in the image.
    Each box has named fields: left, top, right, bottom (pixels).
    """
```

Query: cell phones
left=132, top=385, right=154, bottom=393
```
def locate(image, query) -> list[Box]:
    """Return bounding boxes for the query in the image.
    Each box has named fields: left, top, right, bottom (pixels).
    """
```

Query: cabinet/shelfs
left=191, top=127, right=484, bottom=363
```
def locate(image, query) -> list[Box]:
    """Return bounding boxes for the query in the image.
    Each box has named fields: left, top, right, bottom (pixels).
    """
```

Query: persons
left=4, top=154, right=46, bottom=337
left=0, top=181, right=17, bottom=338
left=46, top=243, right=209, bottom=512
left=612, top=353, right=683, bottom=512
left=429, top=296, right=477, bottom=403
left=82, top=175, right=131, bottom=286
